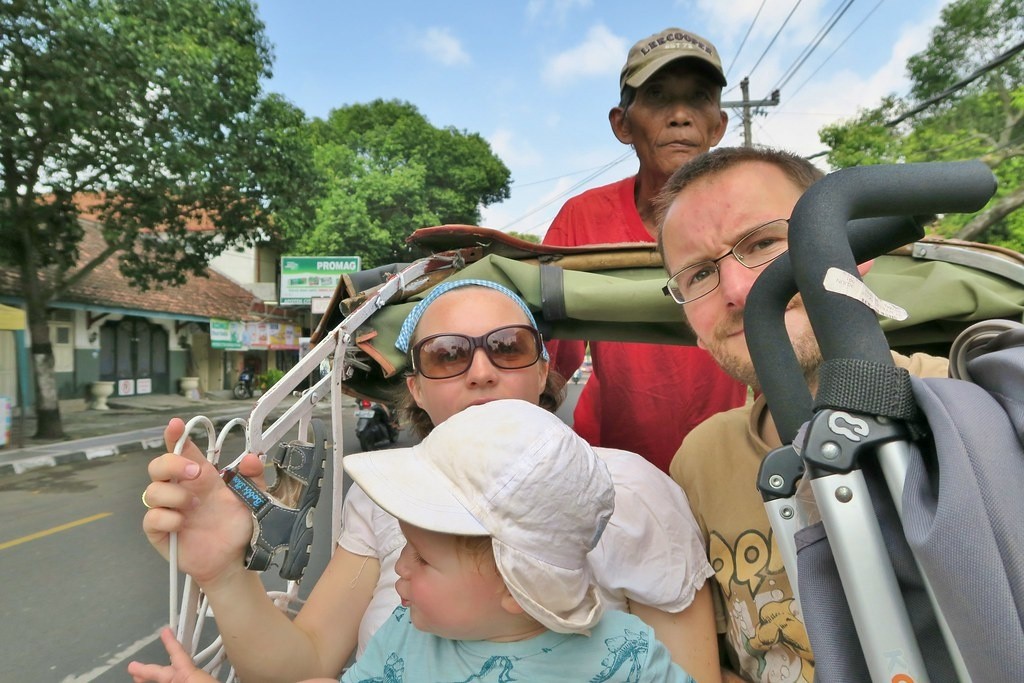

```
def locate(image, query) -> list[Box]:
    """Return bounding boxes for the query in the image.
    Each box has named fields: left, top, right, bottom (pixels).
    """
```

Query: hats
left=620, top=27, right=727, bottom=94
left=342, top=397, right=616, bottom=634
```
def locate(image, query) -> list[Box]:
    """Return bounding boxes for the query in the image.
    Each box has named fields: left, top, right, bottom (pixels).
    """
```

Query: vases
left=181, top=376, right=201, bottom=399
left=89, top=380, right=115, bottom=409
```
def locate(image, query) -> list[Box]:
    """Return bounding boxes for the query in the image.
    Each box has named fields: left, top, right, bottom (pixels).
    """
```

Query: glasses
left=662, top=218, right=790, bottom=306
left=411, top=324, right=543, bottom=379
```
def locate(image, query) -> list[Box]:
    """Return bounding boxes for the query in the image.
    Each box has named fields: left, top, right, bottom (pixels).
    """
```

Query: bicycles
left=235, top=380, right=254, bottom=399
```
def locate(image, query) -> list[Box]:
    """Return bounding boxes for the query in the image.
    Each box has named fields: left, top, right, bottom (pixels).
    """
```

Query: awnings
left=0, top=296, right=211, bottom=334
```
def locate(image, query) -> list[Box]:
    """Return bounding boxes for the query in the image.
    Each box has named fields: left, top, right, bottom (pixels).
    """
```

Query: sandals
left=225, top=418, right=328, bottom=581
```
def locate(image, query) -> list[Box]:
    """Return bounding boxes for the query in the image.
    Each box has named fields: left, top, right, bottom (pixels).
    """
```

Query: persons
left=652, top=146, right=949, bottom=683
left=142, top=284, right=722, bottom=683
left=537, top=28, right=763, bottom=477
left=126, top=399, right=694, bottom=683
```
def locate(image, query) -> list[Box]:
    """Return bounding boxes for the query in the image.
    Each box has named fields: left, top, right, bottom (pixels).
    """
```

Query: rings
left=142, top=490, right=153, bottom=509
